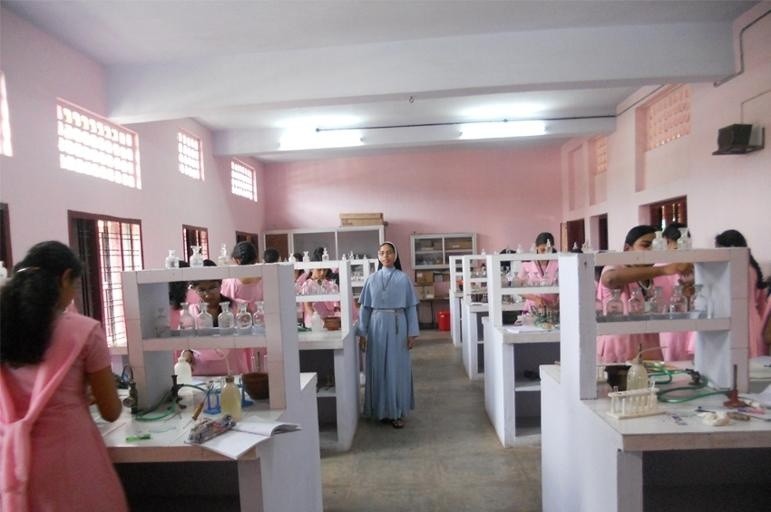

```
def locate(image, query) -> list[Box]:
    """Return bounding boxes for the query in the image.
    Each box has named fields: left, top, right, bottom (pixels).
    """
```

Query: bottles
left=611, top=377, right=660, bottom=414
left=595, top=282, right=712, bottom=320
left=625, top=345, right=646, bottom=397
left=302, top=251, right=310, bottom=263
left=322, top=247, right=330, bottom=262
left=219, top=376, right=243, bottom=423
left=154, top=304, right=173, bottom=337
left=178, top=300, right=264, bottom=336
left=652, top=230, right=667, bottom=249
left=471, top=239, right=589, bottom=325
left=278, top=253, right=296, bottom=263
left=216, top=241, right=231, bottom=268
left=311, top=312, right=321, bottom=334
left=677, top=226, right=692, bottom=249
left=174, top=356, right=192, bottom=386
left=164, top=249, right=182, bottom=269
left=188, top=244, right=204, bottom=267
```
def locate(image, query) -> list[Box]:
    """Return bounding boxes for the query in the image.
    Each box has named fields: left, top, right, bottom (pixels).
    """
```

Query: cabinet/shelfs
left=86, top=261, right=326, bottom=511
left=262, top=223, right=388, bottom=267
left=458, top=253, right=530, bottom=383
left=479, top=252, right=562, bottom=451
left=444, top=251, right=490, bottom=350
left=536, top=245, right=771, bottom=512
left=273, top=260, right=361, bottom=452
left=409, top=233, right=478, bottom=270
left=348, top=257, right=370, bottom=387
left=370, top=259, right=380, bottom=278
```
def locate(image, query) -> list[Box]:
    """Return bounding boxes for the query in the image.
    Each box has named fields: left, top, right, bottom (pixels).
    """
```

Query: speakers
left=718, top=123, right=765, bottom=152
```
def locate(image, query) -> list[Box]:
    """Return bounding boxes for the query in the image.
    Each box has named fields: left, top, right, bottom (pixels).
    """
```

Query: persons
left=516, top=233, right=563, bottom=328
left=358, top=242, right=420, bottom=426
left=168, top=239, right=356, bottom=376
left=0, top=240, right=129, bottom=512
left=594, top=219, right=769, bottom=369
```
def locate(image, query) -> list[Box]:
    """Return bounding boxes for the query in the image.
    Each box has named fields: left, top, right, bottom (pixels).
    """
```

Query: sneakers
left=393, top=417, right=405, bottom=428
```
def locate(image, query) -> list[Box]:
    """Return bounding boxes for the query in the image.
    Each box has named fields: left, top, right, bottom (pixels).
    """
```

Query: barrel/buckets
left=436, top=311, right=450, bottom=331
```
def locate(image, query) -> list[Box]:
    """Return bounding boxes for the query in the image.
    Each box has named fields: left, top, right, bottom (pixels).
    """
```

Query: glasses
left=193, top=286, right=221, bottom=296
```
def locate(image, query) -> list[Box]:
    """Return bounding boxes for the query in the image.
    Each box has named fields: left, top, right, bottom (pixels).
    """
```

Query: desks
left=416, top=297, right=450, bottom=329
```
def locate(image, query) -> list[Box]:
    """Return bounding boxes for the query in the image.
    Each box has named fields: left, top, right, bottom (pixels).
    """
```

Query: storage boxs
left=339, top=212, right=385, bottom=226
left=416, top=270, right=450, bottom=299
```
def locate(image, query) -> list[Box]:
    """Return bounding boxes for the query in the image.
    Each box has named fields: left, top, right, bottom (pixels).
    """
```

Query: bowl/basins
left=241, top=371, right=269, bottom=401
